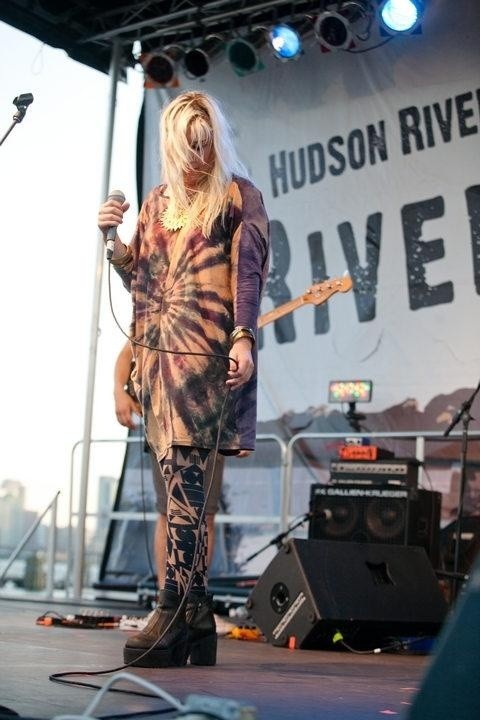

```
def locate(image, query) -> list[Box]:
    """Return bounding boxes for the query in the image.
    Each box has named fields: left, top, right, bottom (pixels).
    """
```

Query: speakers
left=245, top=539, right=445, bottom=652
left=308, top=482, right=443, bottom=563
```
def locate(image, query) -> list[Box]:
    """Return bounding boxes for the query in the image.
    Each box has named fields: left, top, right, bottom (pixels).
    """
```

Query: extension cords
left=182, top=695, right=259, bottom=720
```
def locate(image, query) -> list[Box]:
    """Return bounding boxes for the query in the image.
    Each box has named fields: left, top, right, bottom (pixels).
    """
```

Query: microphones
left=104, top=189, right=125, bottom=266
left=307, top=510, right=333, bottom=520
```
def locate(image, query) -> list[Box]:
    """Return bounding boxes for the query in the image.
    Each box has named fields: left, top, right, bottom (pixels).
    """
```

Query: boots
left=123, top=589, right=218, bottom=668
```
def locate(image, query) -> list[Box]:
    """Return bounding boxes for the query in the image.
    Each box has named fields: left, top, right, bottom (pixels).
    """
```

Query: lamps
left=140, top=0, right=430, bottom=90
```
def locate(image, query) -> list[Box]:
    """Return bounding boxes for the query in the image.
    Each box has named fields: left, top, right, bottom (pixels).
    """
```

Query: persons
left=95, top=91, right=271, bottom=668
